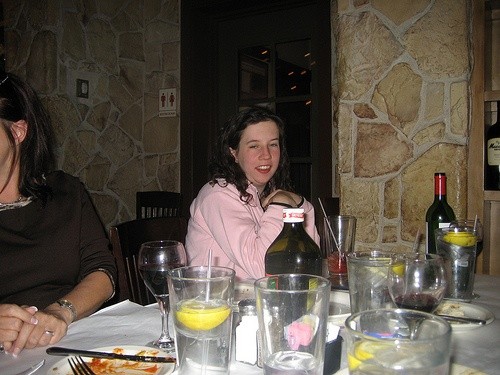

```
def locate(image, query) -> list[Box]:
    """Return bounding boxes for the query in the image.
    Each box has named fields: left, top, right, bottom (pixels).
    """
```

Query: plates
left=46, top=345, right=175, bottom=375
left=431, top=300, right=494, bottom=333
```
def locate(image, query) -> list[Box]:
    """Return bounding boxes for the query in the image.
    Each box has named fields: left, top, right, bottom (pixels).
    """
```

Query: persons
left=186, top=107, right=320, bottom=281
left=0, top=72, right=118, bottom=358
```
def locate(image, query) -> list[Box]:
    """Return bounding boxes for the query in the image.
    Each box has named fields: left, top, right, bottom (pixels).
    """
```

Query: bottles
left=425, top=173, right=455, bottom=257
left=265, top=208, right=322, bottom=354
left=236, top=299, right=258, bottom=365
left=487, top=101, right=500, bottom=190
left=346, top=251, right=406, bottom=316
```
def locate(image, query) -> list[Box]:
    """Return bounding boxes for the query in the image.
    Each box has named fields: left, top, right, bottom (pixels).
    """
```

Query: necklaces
left=18, top=196, right=22, bottom=202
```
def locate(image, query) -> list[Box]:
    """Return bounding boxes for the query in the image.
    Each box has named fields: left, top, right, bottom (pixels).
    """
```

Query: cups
left=434, top=227, right=477, bottom=302
left=167, top=265, right=236, bottom=375
left=324, top=216, right=356, bottom=279
left=254, top=274, right=331, bottom=375
left=345, top=308, right=452, bottom=375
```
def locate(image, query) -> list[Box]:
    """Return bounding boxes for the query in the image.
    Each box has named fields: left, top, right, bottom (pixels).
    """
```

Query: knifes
left=46, top=347, right=176, bottom=363
left=15, top=360, right=44, bottom=375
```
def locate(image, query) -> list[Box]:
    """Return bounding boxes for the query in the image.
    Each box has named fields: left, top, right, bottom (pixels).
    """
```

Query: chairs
left=109, top=191, right=187, bottom=304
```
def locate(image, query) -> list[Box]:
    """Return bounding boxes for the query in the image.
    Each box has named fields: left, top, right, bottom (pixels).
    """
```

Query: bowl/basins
left=328, top=292, right=351, bottom=327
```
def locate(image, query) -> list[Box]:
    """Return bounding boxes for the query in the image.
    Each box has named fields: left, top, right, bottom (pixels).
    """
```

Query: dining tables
left=0, top=274, right=500, bottom=375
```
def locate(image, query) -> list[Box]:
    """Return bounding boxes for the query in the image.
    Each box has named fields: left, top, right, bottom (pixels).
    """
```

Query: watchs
left=54, top=299, right=76, bottom=322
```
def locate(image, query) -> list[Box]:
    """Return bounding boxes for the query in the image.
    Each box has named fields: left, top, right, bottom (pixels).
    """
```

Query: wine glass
left=443, top=221, right=484, bottom=300
left=388, top=254, right=448, bottom=341
left=138, top=241, right=187, bottom=352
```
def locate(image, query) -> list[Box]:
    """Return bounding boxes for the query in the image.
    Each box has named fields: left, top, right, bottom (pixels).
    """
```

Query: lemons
left=391, top=262, right=404, bottom=276
left=347, top=342, right=380, bottom=372
left=443, top=232, right=477, bottom=246
left=177, top=306, right=232, bottom=330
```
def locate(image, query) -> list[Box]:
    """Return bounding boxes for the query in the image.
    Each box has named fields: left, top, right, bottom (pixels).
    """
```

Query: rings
left=44, top=331, right=53, bottom=334
left=0, top=344, right=4, bottom=352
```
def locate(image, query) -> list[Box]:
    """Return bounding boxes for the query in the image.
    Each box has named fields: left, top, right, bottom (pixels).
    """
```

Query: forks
left=68, top=355, right=96, bottom=375
